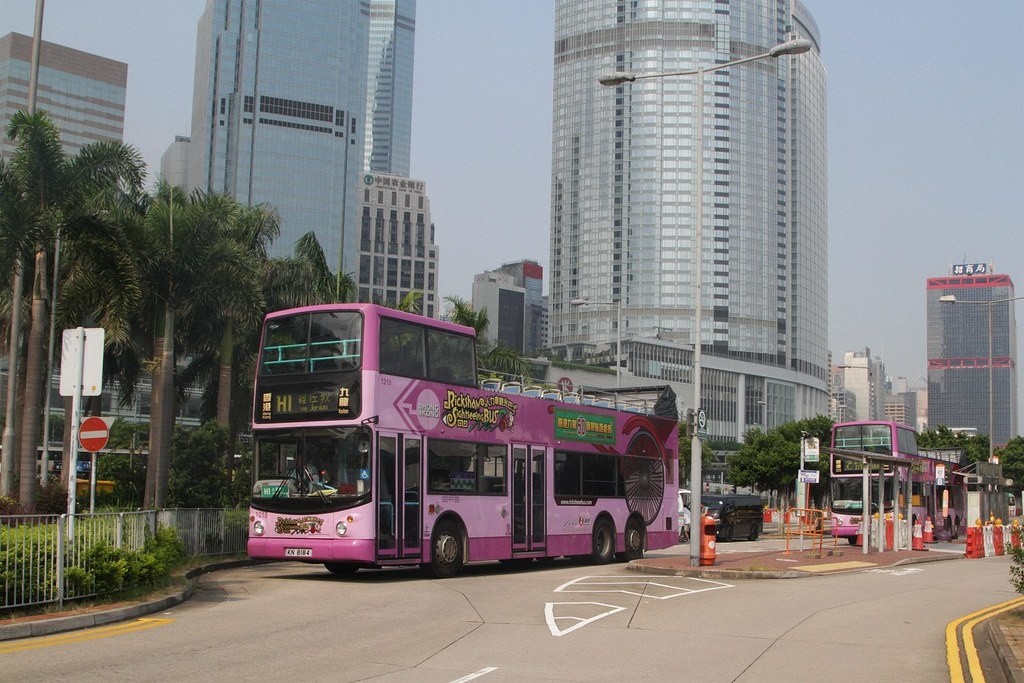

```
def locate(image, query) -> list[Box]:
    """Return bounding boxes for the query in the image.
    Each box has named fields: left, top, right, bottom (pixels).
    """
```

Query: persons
left=287, top=452, right=319, bottom=494
left=684, top=501, right=711, bottom=542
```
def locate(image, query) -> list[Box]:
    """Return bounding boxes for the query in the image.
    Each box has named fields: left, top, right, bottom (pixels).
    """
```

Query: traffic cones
left=912, top=513, right=939, bottom=551
left=856, top=517, right=863, bottom=546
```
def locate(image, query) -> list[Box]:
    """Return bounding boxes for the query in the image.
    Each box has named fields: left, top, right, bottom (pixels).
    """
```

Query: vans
left=687, top=495, right=764, bottom=541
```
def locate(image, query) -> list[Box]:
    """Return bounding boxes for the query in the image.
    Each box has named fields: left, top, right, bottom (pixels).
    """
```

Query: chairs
left=406, top=361, right=423, bottom=380
left=384, top=351, right=402, bottom=372
left=481, top=377, right=653, bottom=414
left=437, top=366, right=455, bottom=382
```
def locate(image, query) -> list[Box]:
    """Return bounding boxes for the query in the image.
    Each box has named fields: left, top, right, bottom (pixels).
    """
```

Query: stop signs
left=78, top=417, right=109, bottom=452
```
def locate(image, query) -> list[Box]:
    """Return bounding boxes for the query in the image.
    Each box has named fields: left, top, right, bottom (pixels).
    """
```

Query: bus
left=830, top=421, right=967, bottom=544
left=247, top=303, right=680, bottom=578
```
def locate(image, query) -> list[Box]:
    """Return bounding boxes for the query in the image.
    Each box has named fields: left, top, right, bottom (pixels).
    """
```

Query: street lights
left=570, top=298, right=622, bottom=390
left=837, top=365, right=878, bottom=422
left=937, top=294, right=1023, bottom=458
left=600, top=39, right=812, bottom=565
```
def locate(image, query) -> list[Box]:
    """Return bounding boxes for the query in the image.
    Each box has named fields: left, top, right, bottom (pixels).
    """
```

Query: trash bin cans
left=700, top=515, right=717, bottom=566
